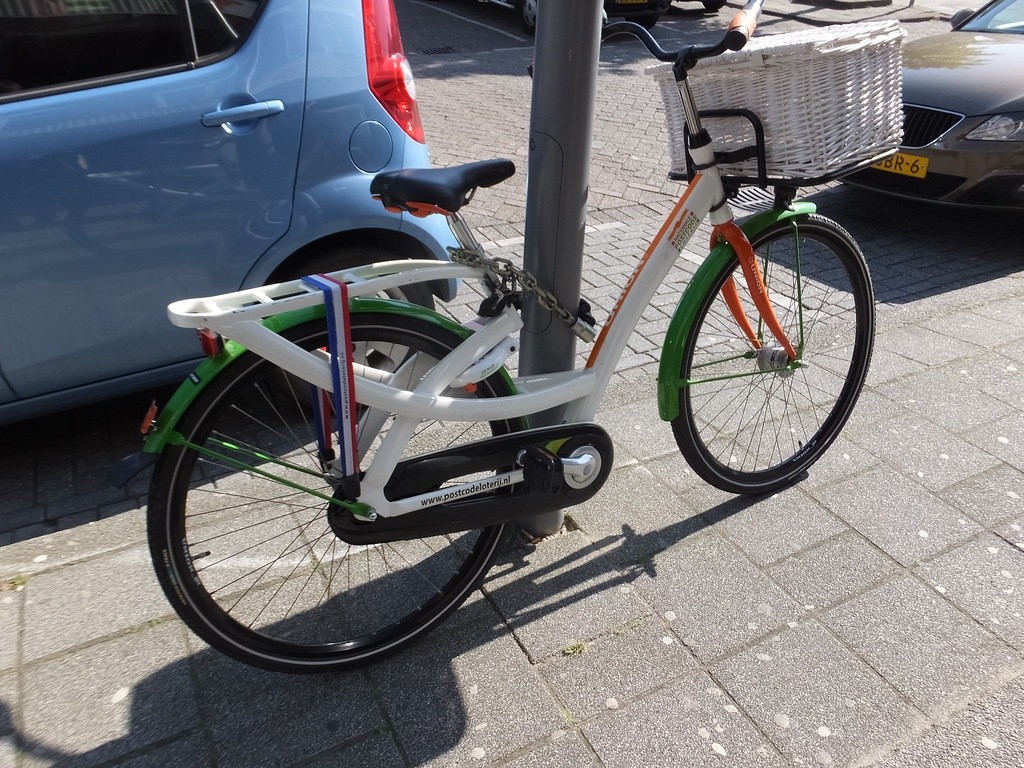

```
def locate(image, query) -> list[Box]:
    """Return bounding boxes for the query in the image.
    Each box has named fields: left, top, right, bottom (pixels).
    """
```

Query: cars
left=826, top=0, right=1024, bottom=210
left=1, top=1, right=463, bottom=423
left=460, top=0, right=673, bottom=39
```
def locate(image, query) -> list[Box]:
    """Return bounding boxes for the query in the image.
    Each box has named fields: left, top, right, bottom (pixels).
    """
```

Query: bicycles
left=132, top=1, right=908, bottom=676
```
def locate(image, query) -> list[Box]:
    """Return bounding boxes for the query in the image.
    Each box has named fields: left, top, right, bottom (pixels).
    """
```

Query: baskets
left=644, top=18, right=908, bottom=179
